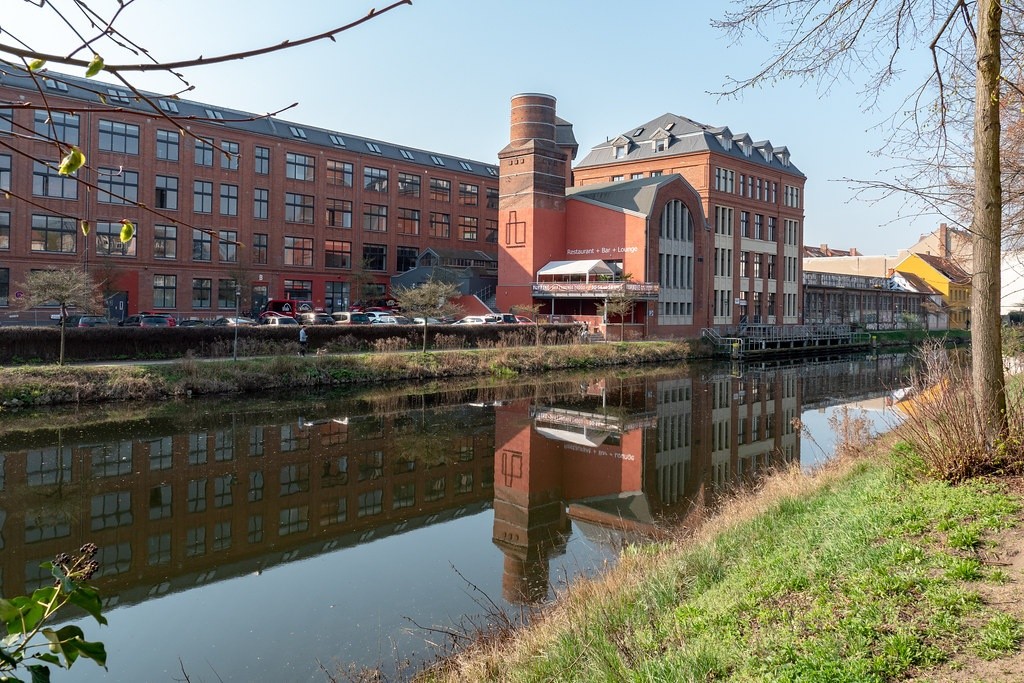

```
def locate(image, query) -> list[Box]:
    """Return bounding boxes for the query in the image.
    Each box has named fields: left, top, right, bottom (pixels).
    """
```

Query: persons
left=296, top=325, right=309, bottom=357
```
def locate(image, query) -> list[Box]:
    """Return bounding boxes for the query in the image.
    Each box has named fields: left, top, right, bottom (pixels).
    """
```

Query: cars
left=140, top=311, right=176, bottom=326
left=371, top=315, right=409, bottom=325
left=451, top=316, right=497, bottom=325
left=180, top=320, right=213, bottom=326
left=364, top=311, right=391, bottom=320
left=413, top=317, right=440, bottom=325
left=296, top=312, right=335, bottom=325
left=123, top=315, right=169, bottom=327
left=263, top=316, right=298, bottom=325
left=515, top=316, right=533, bottom=322
left=486, top=313, right=518, bottom=324
left=213, top=315, right=261, bottom=327
left=58, top=314, right=110, bottom=327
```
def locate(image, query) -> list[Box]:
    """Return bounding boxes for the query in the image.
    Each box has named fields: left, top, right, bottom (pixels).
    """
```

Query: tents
left=535, top=257, right=616, bottom=290
left=533, top=419, right=613, bottom=448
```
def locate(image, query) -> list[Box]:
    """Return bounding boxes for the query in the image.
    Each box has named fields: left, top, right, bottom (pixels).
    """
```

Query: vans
left=262, top=300, right=312, bottom=321
left=331, top=312, right=370, bottom=324
left=348, top=298, right=391, bottom=312
